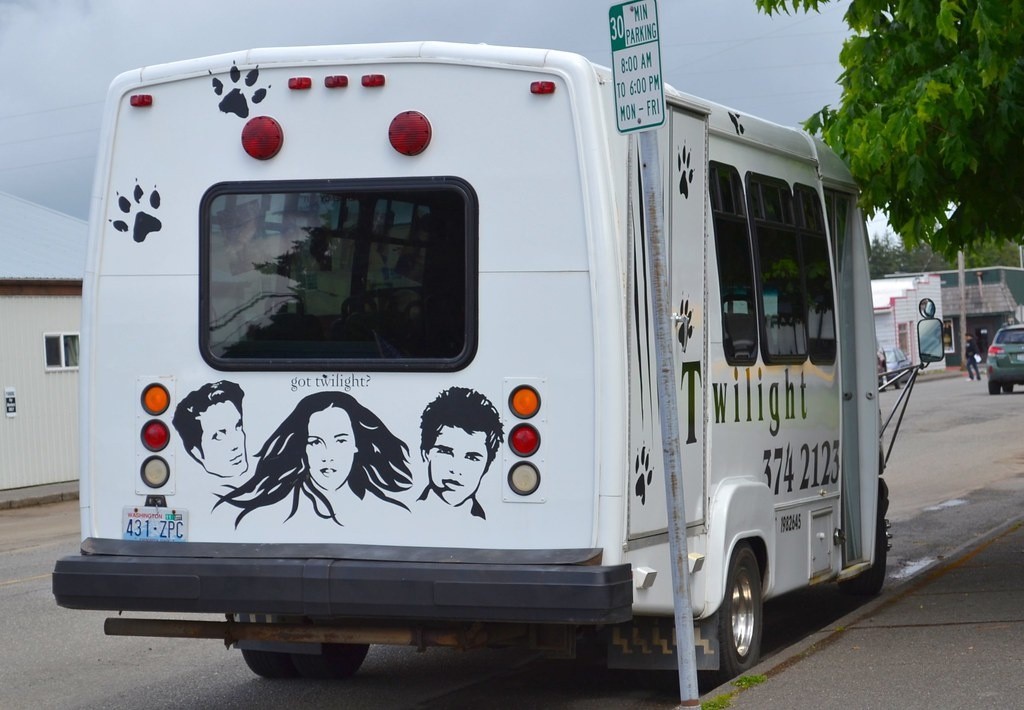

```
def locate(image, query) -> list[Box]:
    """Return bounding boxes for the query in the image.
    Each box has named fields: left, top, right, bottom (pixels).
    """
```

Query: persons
left=964, top=333, right=981, bottom=381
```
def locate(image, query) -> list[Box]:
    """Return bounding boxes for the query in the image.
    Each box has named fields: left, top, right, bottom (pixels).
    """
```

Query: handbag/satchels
left=974, top=353, right=982, bottom=362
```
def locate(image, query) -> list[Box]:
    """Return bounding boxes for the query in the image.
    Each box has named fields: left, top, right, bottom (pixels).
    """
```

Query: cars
left=883, top=348, right=911, bottom=390
left=986, top=325, right=1024, bottom=395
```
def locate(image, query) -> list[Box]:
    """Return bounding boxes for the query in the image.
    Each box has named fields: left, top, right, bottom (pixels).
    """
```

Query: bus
left=56, top=42, right=946, bottom=681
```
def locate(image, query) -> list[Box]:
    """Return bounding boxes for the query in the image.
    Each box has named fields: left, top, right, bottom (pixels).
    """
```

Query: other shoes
left=967, top=379, right=974, bottom=381
